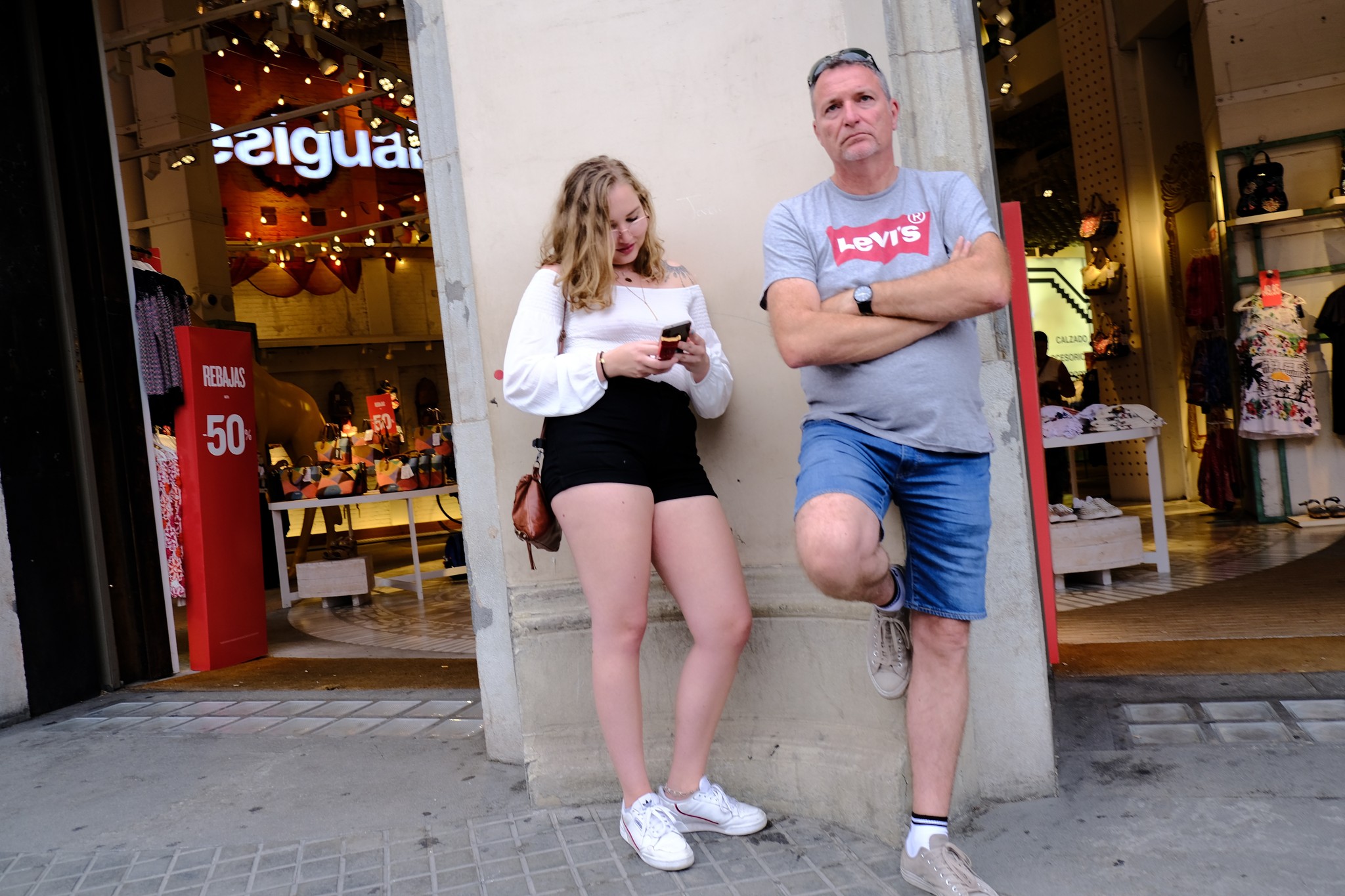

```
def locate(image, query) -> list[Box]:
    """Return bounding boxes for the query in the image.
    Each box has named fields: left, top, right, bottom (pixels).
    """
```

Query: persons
left=759, top=47, right=1013, bottom=896
left=502, top=155, right=768, bottom=870
left=1035, top=330, right=1076, bottom=506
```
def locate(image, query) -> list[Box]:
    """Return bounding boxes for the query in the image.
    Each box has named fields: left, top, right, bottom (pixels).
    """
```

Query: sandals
left=1323, top=496, right=1345, bottom=516
left=1298, top=499, right=1330, bottom=519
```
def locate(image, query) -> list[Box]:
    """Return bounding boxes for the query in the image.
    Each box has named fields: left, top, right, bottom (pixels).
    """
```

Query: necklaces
left=611, top=265, right=659, bottom=321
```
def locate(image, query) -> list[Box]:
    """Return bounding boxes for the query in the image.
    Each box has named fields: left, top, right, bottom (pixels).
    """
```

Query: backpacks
left=1197, top=428, right=1244, bottom=507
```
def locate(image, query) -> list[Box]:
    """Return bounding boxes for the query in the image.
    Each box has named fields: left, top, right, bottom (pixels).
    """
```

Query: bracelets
left=598, top=351, right=611, bottom=382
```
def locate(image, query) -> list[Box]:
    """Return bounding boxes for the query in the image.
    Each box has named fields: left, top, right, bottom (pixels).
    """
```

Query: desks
left=268, top=482, right=457, bottom=608
left=1049, top=514, right=1144, bottom=590
left=1042, top=427, right=1171, bottom=589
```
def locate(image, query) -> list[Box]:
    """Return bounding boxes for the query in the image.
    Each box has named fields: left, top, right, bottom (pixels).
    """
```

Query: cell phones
left=660, top=320, right=693, bottom=357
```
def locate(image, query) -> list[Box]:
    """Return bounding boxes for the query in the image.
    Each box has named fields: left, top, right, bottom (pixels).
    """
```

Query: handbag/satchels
left=351, top=419, right=382, bottom=446
left=315, top=463, right=362, bottom=500
left=274, top=454, right=322, bottom=501
left=1077, top=192, right=1119, bottom=242
left=329, top=462, right=367, bottom=493
left=352, top=443, right=386, bottom=475
left=512, top=475, right=562, bottom=570
left=1236, top=151, right=1288, bottom=217
left=401, top=450, right=432, bottom=489
left=314, top=423, right=351, bottom=466
left=418, top=453, right=445, bottom=488
left=1081, top=245, right=1123, bottom=298
left=374, top=454, right=420, bottom=494
left=413, top=415, right=453, bottom=456
left=1090, top=313, right=1134, bottom=362
left=379, top=419, right=401, bottom=455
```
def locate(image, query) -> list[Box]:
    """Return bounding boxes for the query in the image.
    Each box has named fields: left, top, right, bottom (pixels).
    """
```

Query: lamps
left=141, top=40, right=176, bottom=77
left=1035, top=180, right=1054, bottom=200
left=259, top=0, right=421, bottom=149
left=144, top=146, right=197, bottom=180
left=279, top=222, right=429, bottom=263
left=979, top=0, right=1022, bottom=112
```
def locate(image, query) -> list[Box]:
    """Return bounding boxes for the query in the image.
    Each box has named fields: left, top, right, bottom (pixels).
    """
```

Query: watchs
left=853, top=283, right=875, bottom=318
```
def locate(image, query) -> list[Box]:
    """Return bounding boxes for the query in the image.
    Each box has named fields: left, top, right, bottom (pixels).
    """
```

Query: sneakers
left=1048, top=507, right=1061, bottom=523
left=619, top=792, right=694, bottom=871
left=1052, top=503, right=1078, bottom=521
left=899, top=833, right=998, bottom=896
left=865, top=564, right=911, bottom=700
left=1072, top=497, right=1107, bottom=520
left=658, top=774, right=767, bottom=836
left=1086, top=496, right=1123, bottom=518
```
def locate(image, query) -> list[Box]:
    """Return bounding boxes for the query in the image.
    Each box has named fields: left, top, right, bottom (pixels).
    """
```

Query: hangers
left=152, top=425, right=178, bottom=461
left=1233, top=287, right=1307, bottom=311
left=130, top=245, right=179, bottom=284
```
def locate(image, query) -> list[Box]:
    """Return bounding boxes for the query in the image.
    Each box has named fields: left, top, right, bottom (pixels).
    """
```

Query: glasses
left=808, top=47, right=881, bottom=88
left=611, top=209, right=651, bottom=245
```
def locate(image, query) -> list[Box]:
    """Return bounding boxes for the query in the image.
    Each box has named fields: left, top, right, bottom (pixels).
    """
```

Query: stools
left=295, top=556, right=376, bottom=608
